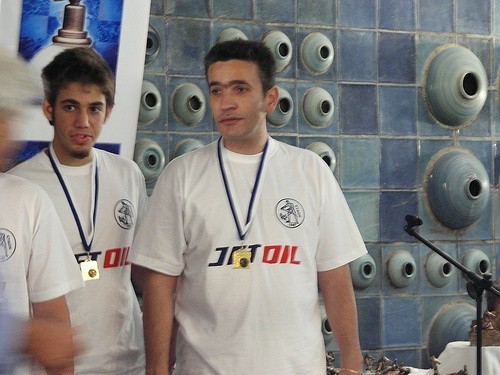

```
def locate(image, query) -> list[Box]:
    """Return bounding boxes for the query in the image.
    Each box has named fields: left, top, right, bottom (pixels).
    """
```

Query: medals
left=79, top=257, right=99, bottom=281
left=231, top=247, right=253, bottom=268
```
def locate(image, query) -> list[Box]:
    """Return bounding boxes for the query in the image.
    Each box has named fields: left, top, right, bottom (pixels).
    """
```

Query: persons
left=4, top=48, right=149, bottom=375
left=126, top=41, right=367, bottom=375
left=0, top=105, right=85, bottom=375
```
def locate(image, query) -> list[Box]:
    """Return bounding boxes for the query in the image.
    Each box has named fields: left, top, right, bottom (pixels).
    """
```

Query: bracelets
left=340, top=367, right=361, bottom=375
